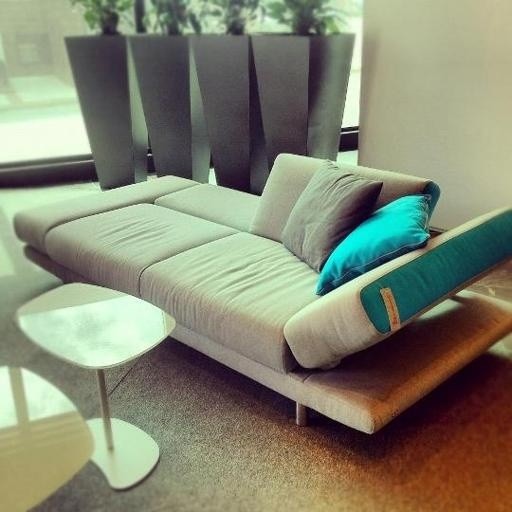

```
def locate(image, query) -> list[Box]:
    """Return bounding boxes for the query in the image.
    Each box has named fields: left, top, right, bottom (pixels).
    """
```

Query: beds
left=11, top=151, right=512, bottom=435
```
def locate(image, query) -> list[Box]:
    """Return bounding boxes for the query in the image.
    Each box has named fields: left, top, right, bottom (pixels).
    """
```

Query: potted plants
left=64, top=0, right=149, bottom=190
left=187, top=4, right=270, bottom=195
left=250, top=0, right=358, bottom=176
left=128, top=1, right=210, bottom=186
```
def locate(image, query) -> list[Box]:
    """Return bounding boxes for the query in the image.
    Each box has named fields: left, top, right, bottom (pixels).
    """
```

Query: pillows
left=281, top=159, right=383, bottom=271
left=316, top=194, right=432, bottom=295
